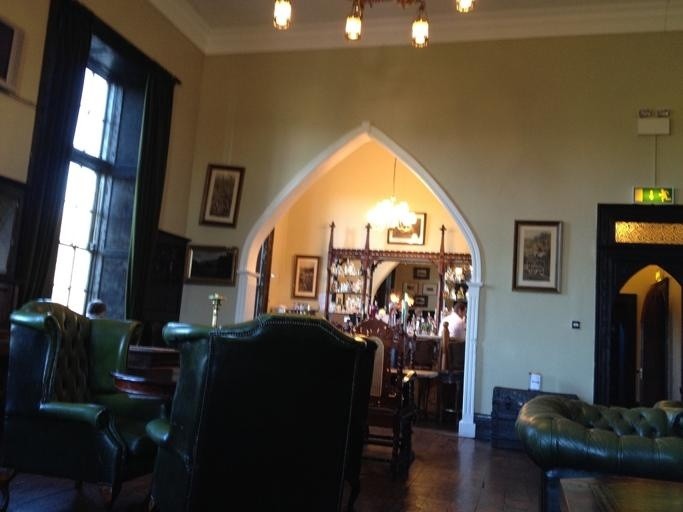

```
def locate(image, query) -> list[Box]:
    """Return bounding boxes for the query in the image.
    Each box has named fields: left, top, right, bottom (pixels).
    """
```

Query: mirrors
left=370, top=258, right=439, bottom=335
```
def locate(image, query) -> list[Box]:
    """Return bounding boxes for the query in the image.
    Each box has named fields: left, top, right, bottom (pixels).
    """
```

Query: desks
left=542, top=470, right=683, bottom=512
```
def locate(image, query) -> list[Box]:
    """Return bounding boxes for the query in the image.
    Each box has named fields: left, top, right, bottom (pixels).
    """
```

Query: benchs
left=490, top=386, right=580, bottom=452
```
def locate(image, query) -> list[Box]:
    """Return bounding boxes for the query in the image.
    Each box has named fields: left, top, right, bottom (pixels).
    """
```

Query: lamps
left=270, top=0, right=473, bottom=49
left=367, top=158, right=418, bottom=228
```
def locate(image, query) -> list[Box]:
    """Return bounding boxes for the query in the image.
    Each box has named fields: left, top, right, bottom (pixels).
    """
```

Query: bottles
left=337, top=298, right=343, bottom=315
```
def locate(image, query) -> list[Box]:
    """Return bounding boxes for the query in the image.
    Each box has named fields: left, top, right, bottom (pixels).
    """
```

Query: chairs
left=385, top=302, right=466, bottom=449
left=513, top=392, right=683, bottom=512
left=152, top=304, right=386, bottom=511
left=1, top=301, right=151, bottom=512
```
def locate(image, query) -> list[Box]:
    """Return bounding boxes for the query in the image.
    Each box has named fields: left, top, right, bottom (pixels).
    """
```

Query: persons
left=86, top=298, right=107, bottom=320
left=438, top=302, right=467, bottom=414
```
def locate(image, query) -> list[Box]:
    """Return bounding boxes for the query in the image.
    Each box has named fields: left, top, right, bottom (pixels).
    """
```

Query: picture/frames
left=511, top=219, right=561, bottom=293
left=183, top=244, right=239, bottom=287
left=293, top=255, right=320, bottom=300
left=199, top=164, right=246, bottom=229
left=0, top=14, right=26, bottom=91
left=387, top=212, right=426, bottom=245
left=402, top=267, right=437, bottom=307
